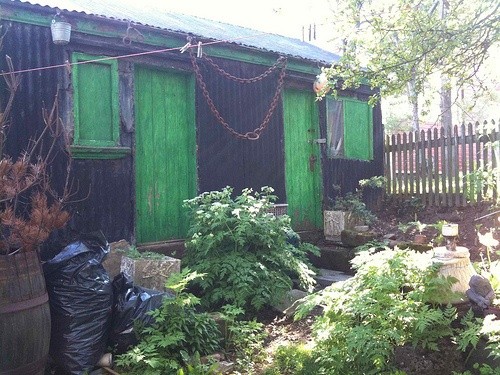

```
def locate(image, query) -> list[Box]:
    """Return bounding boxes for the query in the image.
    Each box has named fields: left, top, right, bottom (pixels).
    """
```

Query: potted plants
left=324, top=183, right=365, bottom=244
left=0, top=157, right=72, bottom=374
left=119, top=248, right=187, bottom=295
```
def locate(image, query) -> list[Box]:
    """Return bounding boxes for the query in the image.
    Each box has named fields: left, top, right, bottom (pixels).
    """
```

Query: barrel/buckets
left=50, top=12, right=72, bottom=45
